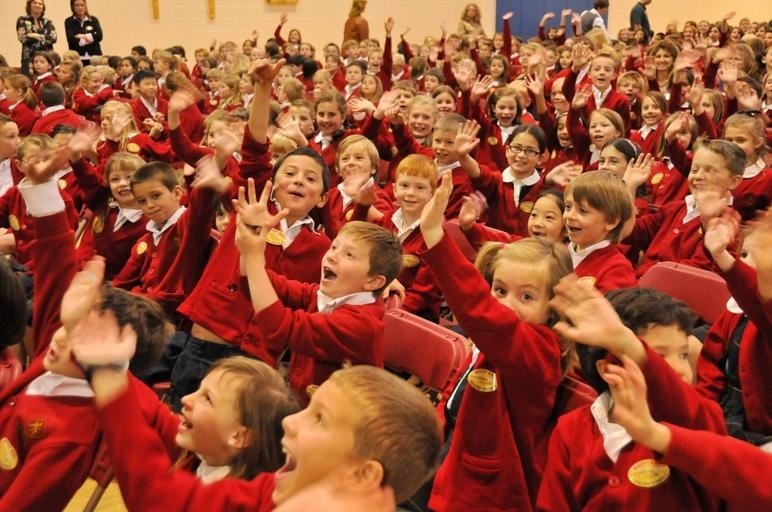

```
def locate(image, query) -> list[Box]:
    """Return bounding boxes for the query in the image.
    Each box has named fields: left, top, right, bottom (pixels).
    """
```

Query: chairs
left=0, top=221, right=732, bottom=512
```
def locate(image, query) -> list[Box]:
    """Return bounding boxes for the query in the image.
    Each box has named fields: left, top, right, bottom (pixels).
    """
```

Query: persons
left=0, top=1, right=771, bottom=512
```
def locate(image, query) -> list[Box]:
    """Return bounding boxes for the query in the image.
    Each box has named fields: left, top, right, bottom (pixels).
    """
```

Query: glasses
left=507, top=145, right=543, bottom=159
